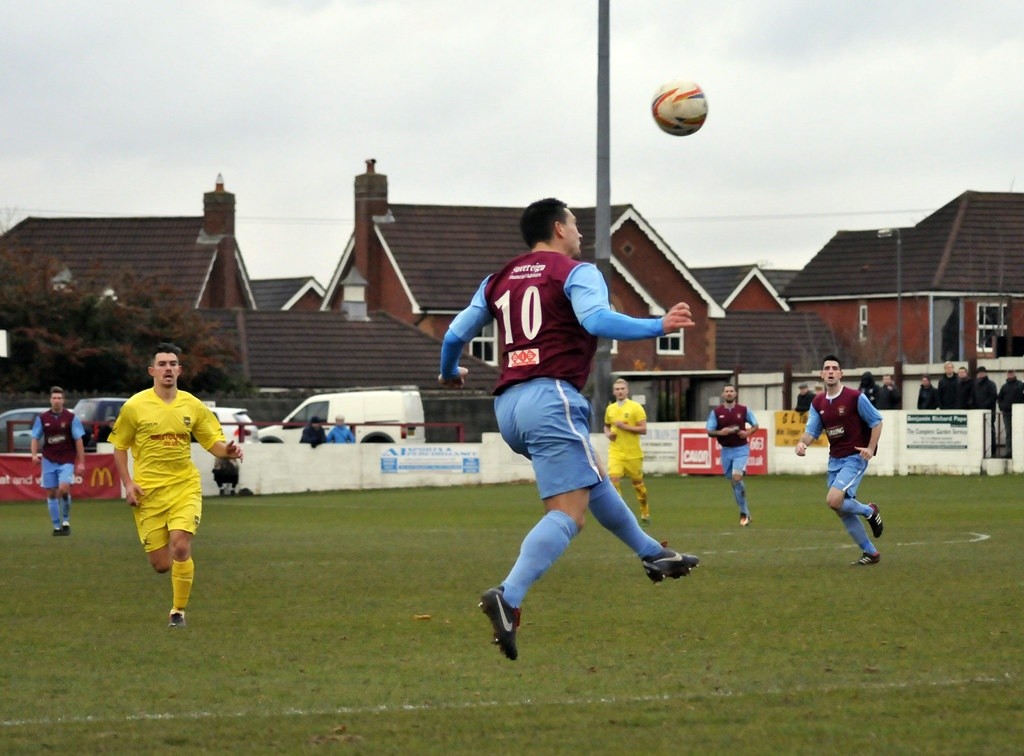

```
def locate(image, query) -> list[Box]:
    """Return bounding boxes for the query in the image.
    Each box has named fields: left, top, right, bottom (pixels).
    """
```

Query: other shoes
left=62, top=525, right=71, bottom=536
left=230, top=490, right=237, bottom=495
left=51, top=527, right=63, bottom=537
left=739, top=513, right=754, bottom=528
left=220, top=489, right=225, bottom=496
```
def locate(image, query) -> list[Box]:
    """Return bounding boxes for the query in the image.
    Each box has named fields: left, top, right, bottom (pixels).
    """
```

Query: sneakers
left=855, top=551, right=881, bottom=565
left=167, top=609, right=188, bottom=628
left=642, top=541, right=702, bottom=583
left=477, top=585, right=522, bottom=660
left=640, top=504, right=650, bottom=520
left=865, top=503, right=883, bottom=538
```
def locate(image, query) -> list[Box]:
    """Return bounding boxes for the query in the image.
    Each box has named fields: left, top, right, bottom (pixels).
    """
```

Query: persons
left=299, top=415, right=327, bottom=448
left=916, top=376, right=939, bottom=409
left=795, top=384, right=817, bottom=413
left=97, top=415, right=116, bottom=442
left=935, top=362, right=997, bottom=458
left=326, top=415, right=356, bottom=444
left=211, top=456, right=240, bottom=496
left=435, top=196, right=700, bottom=662
left=997, top=368, right=1024, bottom=459
left=705, top=384, right=759, bottom=527
left=858, top=372, right=879, bottom=408
left=793, top=355, right=885, bottom=567
left=877, top=374, right=902, bottom=410
left=604, top=378, right=651, bottom=522
left=30, top=387, right=86, bottom=536
left=105, top=342, right=244, bottom=627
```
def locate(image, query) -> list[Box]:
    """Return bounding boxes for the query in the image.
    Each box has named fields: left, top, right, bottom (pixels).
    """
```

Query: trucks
left=258, top=383, right=428, bottom=445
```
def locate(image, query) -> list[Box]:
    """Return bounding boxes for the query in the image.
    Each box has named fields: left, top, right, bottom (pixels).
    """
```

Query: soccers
left=650, top=77, right=709, bottom=137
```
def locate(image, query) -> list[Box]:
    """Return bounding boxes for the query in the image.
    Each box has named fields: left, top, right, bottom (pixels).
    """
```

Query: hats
left=798, top=384, right=808, bottom=389
left=106, top=416, right=117, bottom=422
left=335, top=414, right=347, bottom=420
left=310, top=416, right=322, bottom=424
left=976, top=366, right=987, bottom=373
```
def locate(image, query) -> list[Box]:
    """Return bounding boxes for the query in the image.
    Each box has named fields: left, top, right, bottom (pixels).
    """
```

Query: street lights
left=876, top=227, right=903, bottom=396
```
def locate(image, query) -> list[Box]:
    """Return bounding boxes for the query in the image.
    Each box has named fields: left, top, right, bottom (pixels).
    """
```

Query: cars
left=0, top=396, right=132, bottom=455
left=192, top=406, right=260, bottom=444
